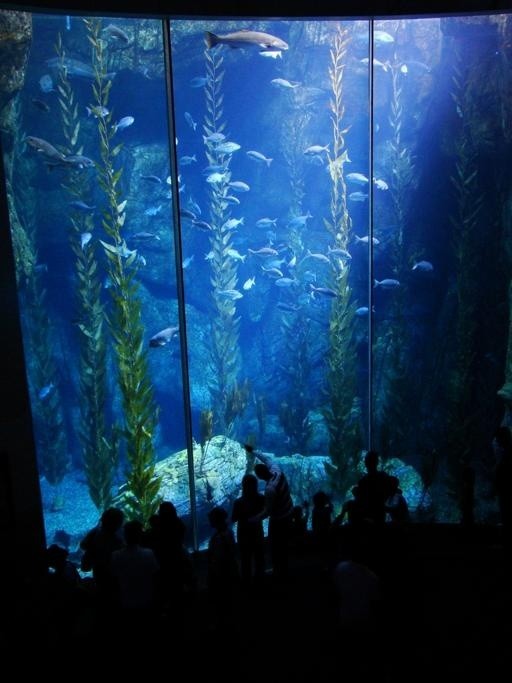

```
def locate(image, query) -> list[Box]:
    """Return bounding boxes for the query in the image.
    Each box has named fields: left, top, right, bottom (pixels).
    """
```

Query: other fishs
left=21, top=99, right=401, bottom=319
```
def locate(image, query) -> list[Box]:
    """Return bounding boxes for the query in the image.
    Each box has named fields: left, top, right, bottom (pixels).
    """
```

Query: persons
left=47, top=452, right=411, bottom=598
